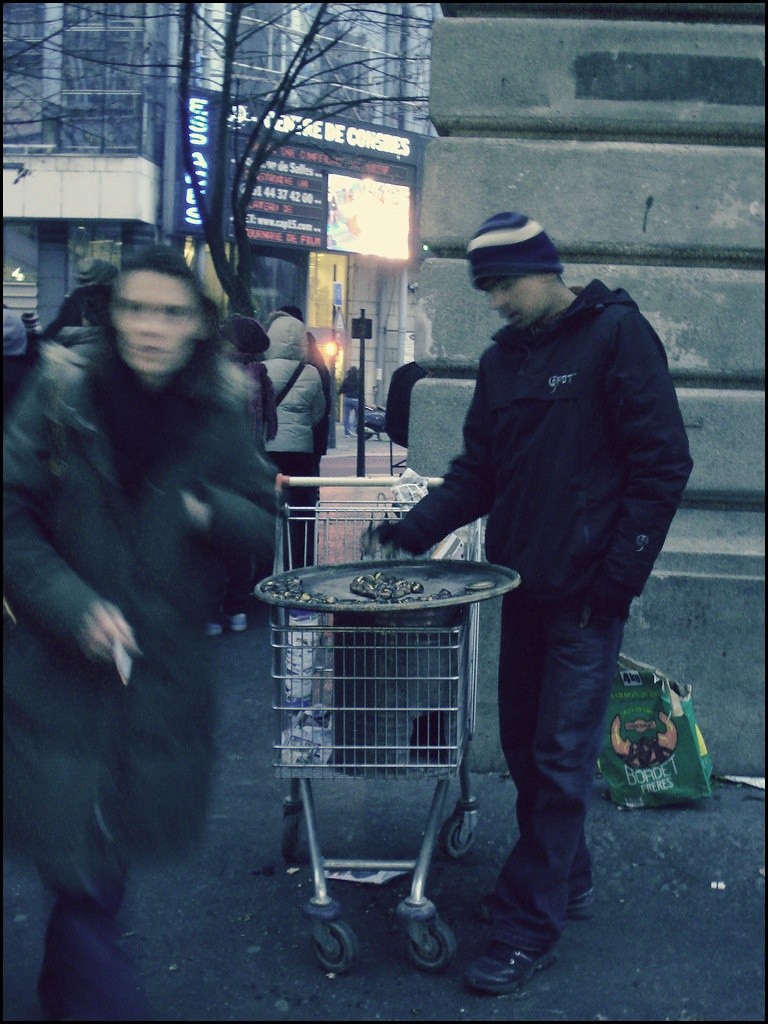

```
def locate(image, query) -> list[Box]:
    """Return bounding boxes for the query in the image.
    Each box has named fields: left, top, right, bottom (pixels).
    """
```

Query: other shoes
left=228, top=613, right=247, bottom=631
left=206, top=623, right=223, bottom=635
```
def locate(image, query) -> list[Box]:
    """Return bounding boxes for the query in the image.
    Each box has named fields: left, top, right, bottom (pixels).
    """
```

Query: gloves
left=380, top=518, right=431, bottom=555
left=586, top=573, right=633, bottom=622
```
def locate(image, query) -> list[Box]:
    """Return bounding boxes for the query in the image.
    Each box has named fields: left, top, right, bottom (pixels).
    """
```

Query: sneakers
left=478, top=873, right=593, bottom=921
left=455, top=940, right=559, bottom=997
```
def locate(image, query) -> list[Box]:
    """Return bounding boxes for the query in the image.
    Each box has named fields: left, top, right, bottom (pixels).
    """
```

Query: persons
left=337, top=366, right=361, bottom=436
left=1, top=254, right=332, bottom=675
left=377, top=213, right=695, bottom=992
left=0, top=243, right=280, bottom=1023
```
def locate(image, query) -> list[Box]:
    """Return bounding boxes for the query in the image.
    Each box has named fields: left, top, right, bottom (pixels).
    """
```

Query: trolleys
left=269, top=472, right=478, bottom=977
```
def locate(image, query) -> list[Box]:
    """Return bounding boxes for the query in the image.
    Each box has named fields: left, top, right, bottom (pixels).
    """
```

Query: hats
left=466, top=211, right=565, bottom=289
left=220, top=312, right=271, bottom=357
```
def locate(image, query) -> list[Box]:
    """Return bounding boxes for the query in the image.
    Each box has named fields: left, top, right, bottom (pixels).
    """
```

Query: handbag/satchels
left=594, top=652, right=714, bottom=810
